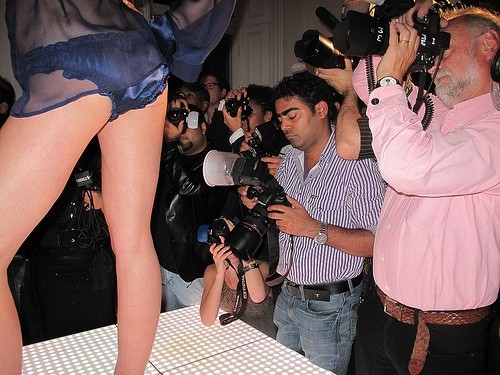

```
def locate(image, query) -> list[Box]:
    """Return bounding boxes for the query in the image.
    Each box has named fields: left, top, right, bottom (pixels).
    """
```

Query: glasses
left=201, top=83, right=221, bottom=89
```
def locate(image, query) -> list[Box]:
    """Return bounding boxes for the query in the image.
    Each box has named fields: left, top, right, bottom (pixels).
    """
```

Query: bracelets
left=243, top=262, right=259, bottom=273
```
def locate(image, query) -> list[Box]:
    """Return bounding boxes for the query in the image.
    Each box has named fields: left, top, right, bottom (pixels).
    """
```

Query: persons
left=301, top=51, right=375, bottom=160
left=1, top=74, right=289, bottom=313
left=200, top=218, right=278, bottom=327
left=348, top=0, right=500, bottom=374
left=238, top=70, right=387, bottom=375
left=1, top=0, right=237, bottom=375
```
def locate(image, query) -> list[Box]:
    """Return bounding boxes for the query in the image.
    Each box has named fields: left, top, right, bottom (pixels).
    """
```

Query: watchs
left=314, top=221, right=328, bottom=244
left=376, top=76, right=401, bottom=88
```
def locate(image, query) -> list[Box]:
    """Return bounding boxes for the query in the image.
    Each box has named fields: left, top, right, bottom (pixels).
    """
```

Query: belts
left=286, top=272, right=363, bottom=302
left=375, top=288, right=490, bottom=375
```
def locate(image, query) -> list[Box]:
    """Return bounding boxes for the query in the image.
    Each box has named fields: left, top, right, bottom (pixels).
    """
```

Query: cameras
left=166, top=94, right=292, bottom=261
left=333, top=0, right=451, bottom=73
left=294, top=30, right=361, bottom=73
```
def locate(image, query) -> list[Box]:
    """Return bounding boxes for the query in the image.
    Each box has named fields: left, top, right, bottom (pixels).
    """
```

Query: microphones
left=74, top=159, right=95, bottom=191
left=315, top=6, right=340, bottom=30
